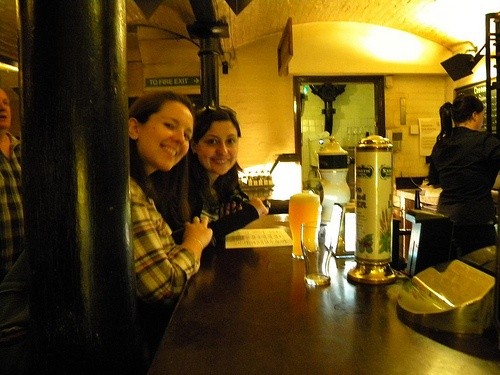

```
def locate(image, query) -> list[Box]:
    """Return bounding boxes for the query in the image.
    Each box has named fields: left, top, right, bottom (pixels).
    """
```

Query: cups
left=300, top=221, right=334, bottom=286
left=288, top=195, right=321, bottom=259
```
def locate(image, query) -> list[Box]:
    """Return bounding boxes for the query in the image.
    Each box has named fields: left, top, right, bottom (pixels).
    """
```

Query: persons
left=0, top=88, right=25, bottom=283
left=0, top=89, right=213, bottom=375
left=155, top=105, right=269, bottom=256
left=429, top=94, right=500, bottom=264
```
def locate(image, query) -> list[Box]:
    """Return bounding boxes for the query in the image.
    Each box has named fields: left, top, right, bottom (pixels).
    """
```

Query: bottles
left=346, top=135, right=396, bottom=285
left=240, top=170, right=273, bottom=187
left=318, top=137, right=351, bottom=208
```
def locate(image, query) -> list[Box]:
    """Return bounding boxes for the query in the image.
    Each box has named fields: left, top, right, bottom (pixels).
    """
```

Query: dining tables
left=151, top=211, right=500, bottom=374
